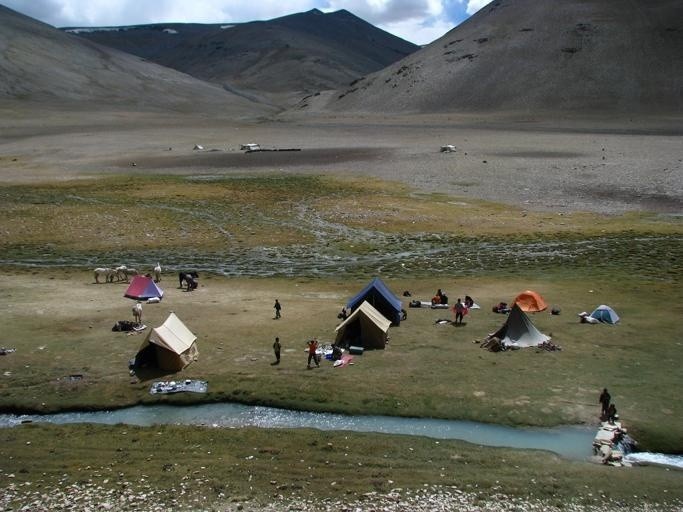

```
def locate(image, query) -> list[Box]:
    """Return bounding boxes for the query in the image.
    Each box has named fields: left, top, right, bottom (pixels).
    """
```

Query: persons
left=273, top=299, right=281, bottom=319
left=330, top=343, right=342, bottom=361
left=453, top=298, right=465, bottom=324
left=305, top=340, right=319, bottom=368
left=605, top=403, right=617, bottom=424
left=491, top=302, right=507, bottom=314
left=272, top=337, right=281, bottom=361
left=598, top=387, right=610, bottom=418
left=434, top=288, right=448, bottom=306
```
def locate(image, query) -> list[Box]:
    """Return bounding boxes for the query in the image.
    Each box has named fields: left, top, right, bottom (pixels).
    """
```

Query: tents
left=122, top=277, right=162, bottom=299
left=589, top=305, right=621, bottom=325
left=127, top=311, right=199, bottom=374
left=330, top=300, right=390, bottom=352
left=512, top=289, right=546, bottom=313
left=481, top=301, right=550, bottom=349
left=344, top=278, right=402, bottom=328
left=192, top=144, right=203, bottom=150
left=240, top=142, right=260, bottom=151
left=439, top=144, right=457, bottom=152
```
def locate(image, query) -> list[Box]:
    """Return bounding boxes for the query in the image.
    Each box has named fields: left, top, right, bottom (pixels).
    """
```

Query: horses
left=152, top=262, right=164, bottom=283
left=178, top=270, right=199, bottom=289
left=94, top=265, right=139, bottom=285
left=131, top=303, right=142, bottom=321
